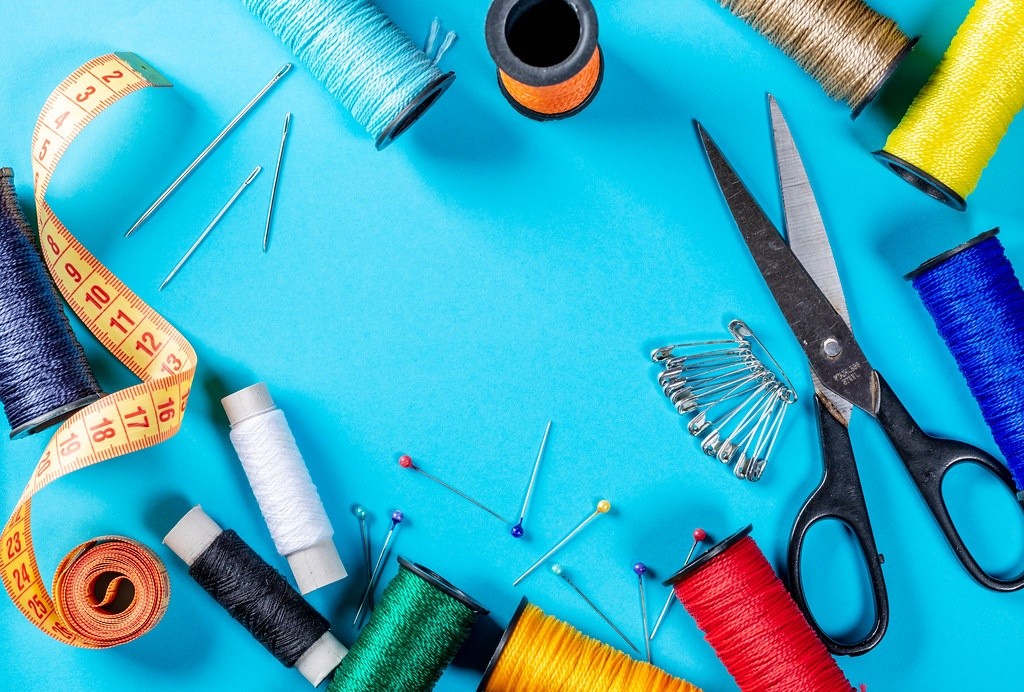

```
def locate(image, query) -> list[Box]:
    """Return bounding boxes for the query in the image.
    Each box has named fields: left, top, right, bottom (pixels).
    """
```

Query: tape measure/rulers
left=1, top=52, right=202, bottom=650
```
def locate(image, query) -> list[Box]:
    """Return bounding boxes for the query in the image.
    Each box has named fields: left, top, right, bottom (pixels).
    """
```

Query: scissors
left=688, top=88, right=1024, bottom=657
